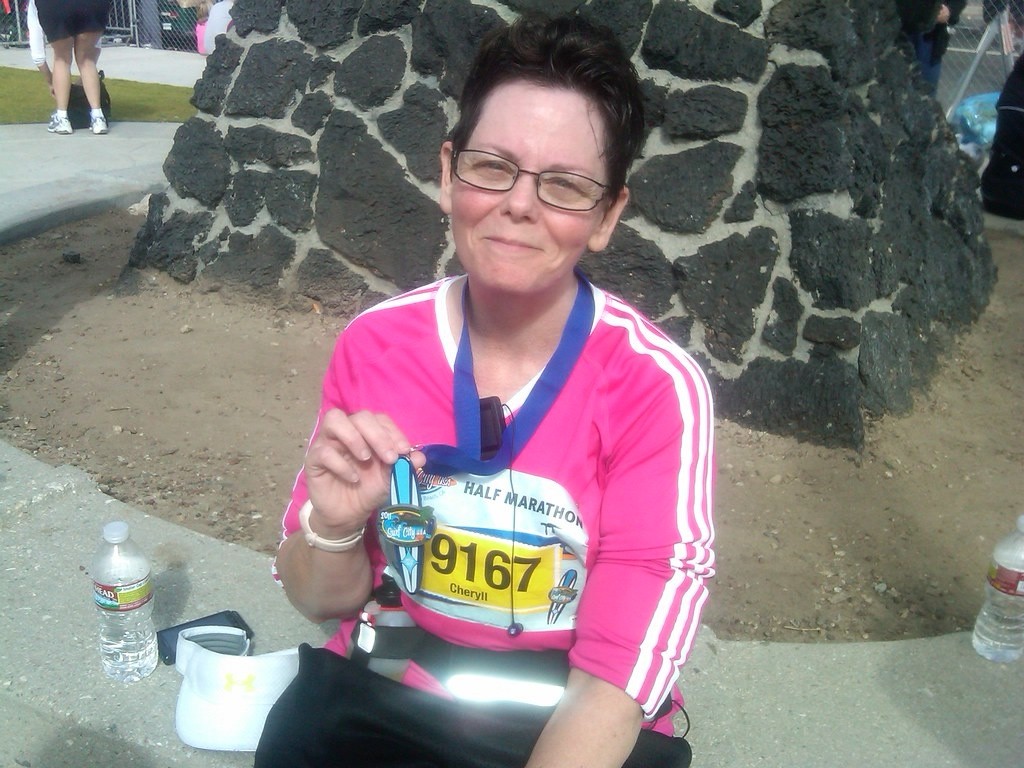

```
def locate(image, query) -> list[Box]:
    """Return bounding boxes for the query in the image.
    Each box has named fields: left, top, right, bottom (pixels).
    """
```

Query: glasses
left=451, top=149, right=617, bottom=212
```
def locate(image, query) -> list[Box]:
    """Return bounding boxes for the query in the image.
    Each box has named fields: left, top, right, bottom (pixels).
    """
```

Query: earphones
left=508, top=622, right=523, bottom=636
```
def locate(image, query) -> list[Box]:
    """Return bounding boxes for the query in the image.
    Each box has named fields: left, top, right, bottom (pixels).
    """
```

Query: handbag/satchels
left=67, top=70, right=111, bottom=130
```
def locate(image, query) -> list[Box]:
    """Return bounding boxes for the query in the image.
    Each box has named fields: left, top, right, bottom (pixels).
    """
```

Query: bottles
left=92, top=522, right=159, bottom=683
left=971, top=514, right=1024, bottom=663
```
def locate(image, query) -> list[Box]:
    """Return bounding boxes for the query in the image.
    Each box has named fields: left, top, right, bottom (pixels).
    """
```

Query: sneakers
left=48, top=113, right=73, bottom=134
left=89, top=112, right=107, bottom=133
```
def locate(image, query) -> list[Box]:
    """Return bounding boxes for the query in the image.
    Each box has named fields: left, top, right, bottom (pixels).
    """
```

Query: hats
left=175, top=625, right=300, bottom=754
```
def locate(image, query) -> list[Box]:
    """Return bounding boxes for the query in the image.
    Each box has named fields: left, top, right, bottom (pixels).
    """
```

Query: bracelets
left=299, top=500, right=365, bottom=552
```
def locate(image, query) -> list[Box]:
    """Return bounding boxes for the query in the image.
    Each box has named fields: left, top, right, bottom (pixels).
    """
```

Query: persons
left=897, top=0, right=966, bottom=93
left=27, top=0, right=112, bottom=134
left=256, top=16, right=715, bottom=768
left=194, top=0, right=234, bottom=57
